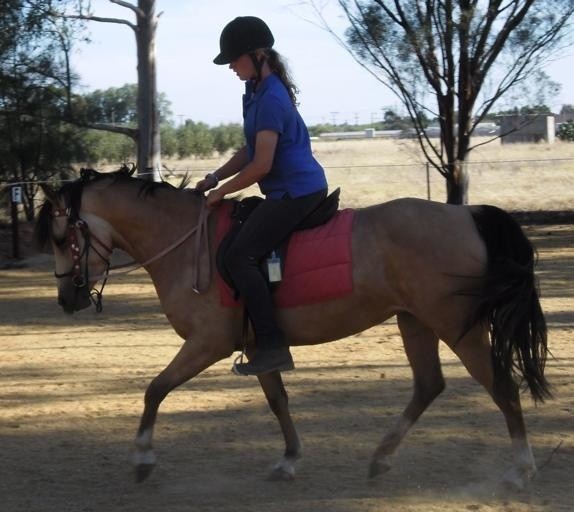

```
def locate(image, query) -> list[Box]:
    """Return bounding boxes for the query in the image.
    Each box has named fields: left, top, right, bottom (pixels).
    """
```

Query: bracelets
left=205, top=173, right=219, bottom=187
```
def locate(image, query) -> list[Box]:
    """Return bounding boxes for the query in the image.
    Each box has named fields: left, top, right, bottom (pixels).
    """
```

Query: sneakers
left=232, top=345, right=295, bottom=376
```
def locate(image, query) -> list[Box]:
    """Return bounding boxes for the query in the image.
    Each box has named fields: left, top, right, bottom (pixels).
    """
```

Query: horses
left=31, top=163, right=558, bottom=493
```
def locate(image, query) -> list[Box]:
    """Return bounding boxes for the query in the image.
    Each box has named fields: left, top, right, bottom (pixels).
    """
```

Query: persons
left=191, top=15, right=335, bottom=378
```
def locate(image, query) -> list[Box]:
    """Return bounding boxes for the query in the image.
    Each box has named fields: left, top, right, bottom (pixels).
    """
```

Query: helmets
left=213, top=16, right=274, bottom=64
